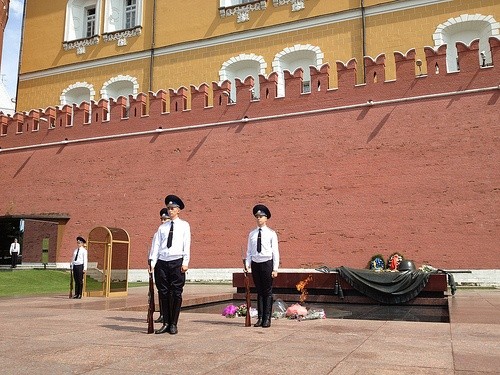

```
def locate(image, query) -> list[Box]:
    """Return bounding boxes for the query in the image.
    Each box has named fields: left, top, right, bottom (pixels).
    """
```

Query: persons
left=148, top=208, right=170, bottom=323
left=72, top=236, right=88, bottom=299
left=9, top=237, right=21, bottom=268
left=243, top=205, right=279, bottom=327
left=151, top=194, right=190, bottom=334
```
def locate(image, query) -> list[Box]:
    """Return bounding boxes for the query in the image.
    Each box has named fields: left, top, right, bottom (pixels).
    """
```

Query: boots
left=155, top=298, right=182, bottom=335
left=154, top=293, right=163, bottom=323
left=254, top=295, right=273, bottom=328
left=73, top=284, right=83, bottom=299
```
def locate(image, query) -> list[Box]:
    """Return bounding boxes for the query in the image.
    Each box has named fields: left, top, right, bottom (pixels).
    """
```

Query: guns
left=243, top=259, right=251, bottom=327
left=147, top=259, right=155, bottom=334
left=69, top=265, right=73, bottom=299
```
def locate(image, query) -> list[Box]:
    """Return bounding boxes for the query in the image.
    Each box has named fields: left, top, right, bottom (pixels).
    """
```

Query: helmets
left=397, top=260, right=416, bottom=271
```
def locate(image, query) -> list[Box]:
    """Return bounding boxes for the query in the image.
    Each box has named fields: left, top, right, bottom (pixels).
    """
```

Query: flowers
left=222, top=298, right=326, bottom=323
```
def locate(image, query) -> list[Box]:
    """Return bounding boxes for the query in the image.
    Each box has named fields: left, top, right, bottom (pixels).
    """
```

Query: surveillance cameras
left=480, top=50, right=486, bottom=58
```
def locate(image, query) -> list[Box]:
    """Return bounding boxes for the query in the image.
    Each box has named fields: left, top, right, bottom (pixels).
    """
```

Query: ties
left=167, top=221, right=174, bottom=248
left=74, top=248, right=79, bottom=261
left=257, top=229, right=262, bottom=253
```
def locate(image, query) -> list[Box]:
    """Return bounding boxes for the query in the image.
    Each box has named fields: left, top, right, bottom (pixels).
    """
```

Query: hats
left=159, top=208, right=170, bottom=218
left=76, top=236, right=86, bottom=244
left=252, top=204, right=271, bottom=219
left=164, top=195, right=185, bottom=210
